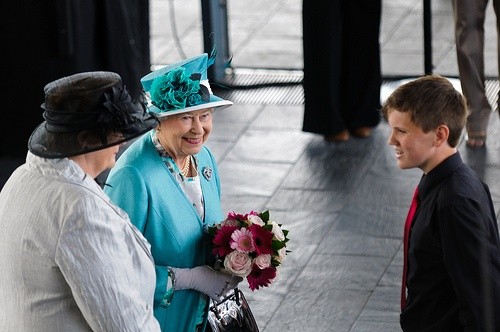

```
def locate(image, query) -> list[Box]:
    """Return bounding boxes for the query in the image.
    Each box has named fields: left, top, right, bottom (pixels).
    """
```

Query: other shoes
left=354, top=127, right=372, bottom=137
left=467, top=130, right=486, bottom=149
left=325, top=129, right=349, bottom=142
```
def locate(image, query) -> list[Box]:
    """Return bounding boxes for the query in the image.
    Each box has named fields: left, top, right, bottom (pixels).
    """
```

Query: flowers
left=207, top=209, right=292, bottom=292
left=149, top=67, right=211, bottom=111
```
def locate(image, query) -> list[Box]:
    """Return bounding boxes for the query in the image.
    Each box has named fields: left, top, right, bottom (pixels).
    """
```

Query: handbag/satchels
left=206, top=287, right=260, bottom=332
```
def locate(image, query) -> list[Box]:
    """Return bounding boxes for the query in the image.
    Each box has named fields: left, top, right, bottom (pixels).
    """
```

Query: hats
left=139, top=52, right=234, bottom=117
left=26, top=71, right=160, bottom=158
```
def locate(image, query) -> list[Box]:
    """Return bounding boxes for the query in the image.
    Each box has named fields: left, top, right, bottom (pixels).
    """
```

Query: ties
left=400, top=185, right=421, bottom=313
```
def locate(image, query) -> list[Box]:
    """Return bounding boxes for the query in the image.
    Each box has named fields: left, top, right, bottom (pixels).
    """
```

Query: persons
left=103, top=54, right=243, bottom=332
left=452, top=0, right=500, bottom=147
left=0, top=72, right=161, bottom=332
left=302, top=0, right=382, bottom=143
left=383, top=74, right=500, bottom=332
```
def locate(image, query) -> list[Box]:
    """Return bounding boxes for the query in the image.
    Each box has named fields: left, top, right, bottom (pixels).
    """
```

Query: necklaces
left=154, top=129, right=190, bottom=177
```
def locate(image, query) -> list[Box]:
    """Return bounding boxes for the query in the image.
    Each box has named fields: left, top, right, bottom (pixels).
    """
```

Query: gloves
left=174, top=265, right=243, bottom=302
